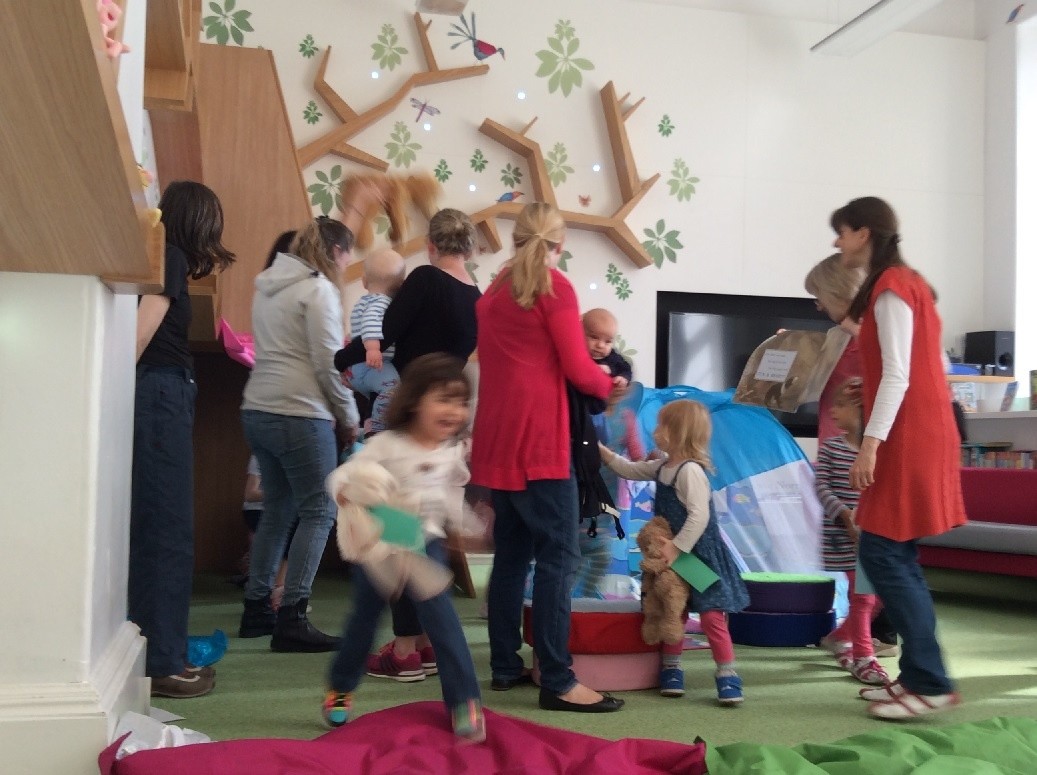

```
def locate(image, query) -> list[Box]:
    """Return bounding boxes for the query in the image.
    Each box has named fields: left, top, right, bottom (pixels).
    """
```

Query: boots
left=238, top=591, right=275, bottom=638
left=270, top=597, right=342, bottom=653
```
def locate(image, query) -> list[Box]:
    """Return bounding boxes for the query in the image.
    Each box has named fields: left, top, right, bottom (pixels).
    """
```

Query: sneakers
left=870, top=689, right=958, bottom=719
left=322, top=690, right=351, bottom=728
left=183, top=663, right=216, bottom=679
left=450, top=697, right=486, bottom=742
left=659, top=669, right=685, bottom=695
left=850, top=656, right=889, bottom=683
left=859, top=680, right=907, bottom=703
left=822, top=634, right=854, bottom=670
left=145, top=668, right=215, bottom=698
left=713, top=672, right=744, bottom=703
left=365, top=640, right=425, bottom=683
left=415, top=645, right=438, bottom=677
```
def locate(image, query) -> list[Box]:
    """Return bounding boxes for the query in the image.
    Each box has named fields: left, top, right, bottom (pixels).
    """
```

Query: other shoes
left=490, top=667, right=536, bottom=691
left=832, top=638, right=898, bottom=657
left=538, top=687, right=624, bottom=713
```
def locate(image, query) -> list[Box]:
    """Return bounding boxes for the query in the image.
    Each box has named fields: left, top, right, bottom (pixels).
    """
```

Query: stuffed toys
left=337, top=473, right=455, bottom=601
left=338, top=174, right=439, bottom=247
left=634, top=518, right=689, bottom=645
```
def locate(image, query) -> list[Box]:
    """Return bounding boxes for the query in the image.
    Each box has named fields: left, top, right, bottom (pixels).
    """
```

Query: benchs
left=915, top=468, right=1037, bottom=601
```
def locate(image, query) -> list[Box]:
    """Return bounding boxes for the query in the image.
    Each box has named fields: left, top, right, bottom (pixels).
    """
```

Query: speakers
left=964, top=329, right=1015, bottom=376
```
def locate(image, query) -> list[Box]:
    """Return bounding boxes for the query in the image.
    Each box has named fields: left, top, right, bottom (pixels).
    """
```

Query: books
left=1000, top=381, right=1018, bottom=412
left=960, top=443, right=1034, bottom=469
left=1029, top=370, right=1037, bottom=410
left=950, top=383, right=977, bottom=412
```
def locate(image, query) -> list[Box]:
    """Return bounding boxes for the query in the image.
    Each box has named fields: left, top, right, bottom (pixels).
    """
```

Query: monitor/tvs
left=657, top=287, right=837, bottom=438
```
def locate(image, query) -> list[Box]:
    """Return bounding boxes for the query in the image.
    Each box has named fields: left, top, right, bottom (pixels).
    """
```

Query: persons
left=129, top=180, right=236, bottom=698
left=778, top=255, right=901, bottom=658
left=814, top=377, right=890, bottom=685
left=598, top=398, right=750, bottom=707
left=347, top=249, right=406, bottom=437
left=830, top=196, right=973, bottom=720
left=477, top=200, right=625, bottom=712
left=260, top=231, right=314, bottom=612
left=238, top=210, right=360, bottom=653
left=580, top=401, right=660, bottom=600
left=334, top=208, right=481, bottom=682
left=324, top=358, right=486, bottom=745
left=582, top=308, right=632, bottom=395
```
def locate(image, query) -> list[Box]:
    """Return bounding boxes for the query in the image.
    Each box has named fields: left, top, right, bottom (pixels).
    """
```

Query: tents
left=528, top=384, right=851, bottom=618
